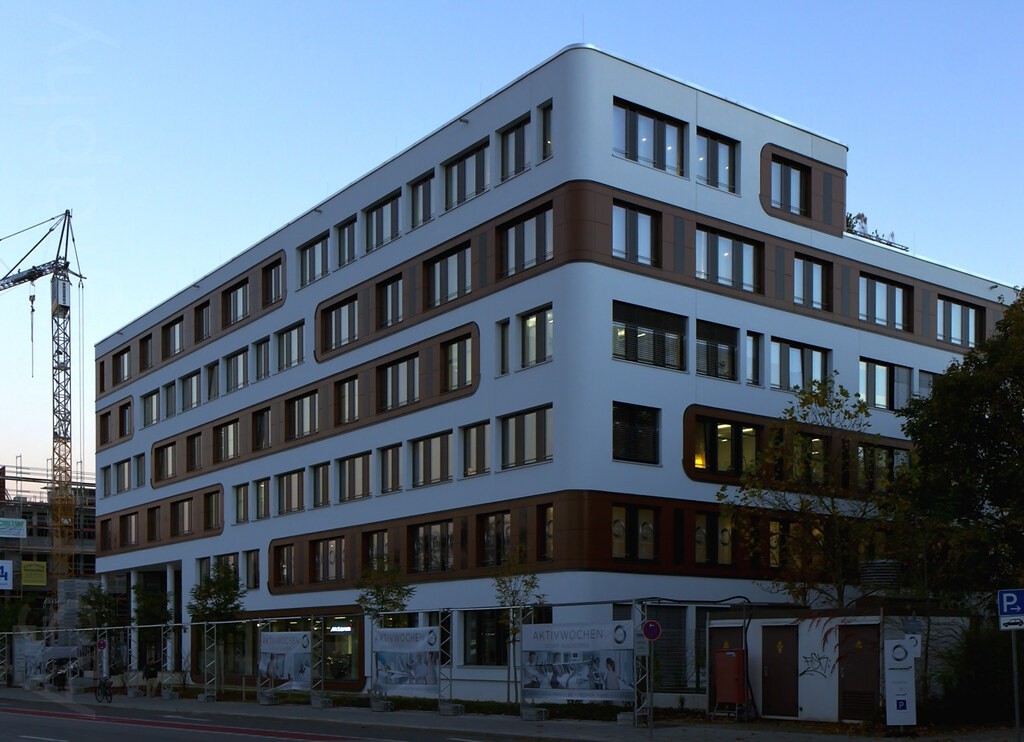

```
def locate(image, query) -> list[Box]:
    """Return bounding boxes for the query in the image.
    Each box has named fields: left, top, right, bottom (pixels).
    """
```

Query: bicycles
left=93, top=677, right=113, bottom=703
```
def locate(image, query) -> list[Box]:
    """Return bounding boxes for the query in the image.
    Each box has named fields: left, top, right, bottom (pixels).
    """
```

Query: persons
left=588, top=658, right=619, bottom=690
left=55, top=664, right=66, bottom=691
left=267, top=653, right=275, bottom=678
left=406, top=651, right=437, bottom=685
left=524, top=651, right=540, bottom=688
left=551, top=653, right=565, bottom=688
left=155, top=648, right=161, bottom=671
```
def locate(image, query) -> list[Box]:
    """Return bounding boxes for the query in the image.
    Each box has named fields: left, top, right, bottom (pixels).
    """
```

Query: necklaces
left=147, top=657, right=157, bottom=697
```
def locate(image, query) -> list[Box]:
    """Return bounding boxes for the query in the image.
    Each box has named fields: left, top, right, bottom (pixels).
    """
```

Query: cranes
left=1, top=209, right=88, bottom=693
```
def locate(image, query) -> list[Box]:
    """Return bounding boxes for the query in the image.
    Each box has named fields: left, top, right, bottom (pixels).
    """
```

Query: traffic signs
left=998, top=589, right=1024, bottom=631
